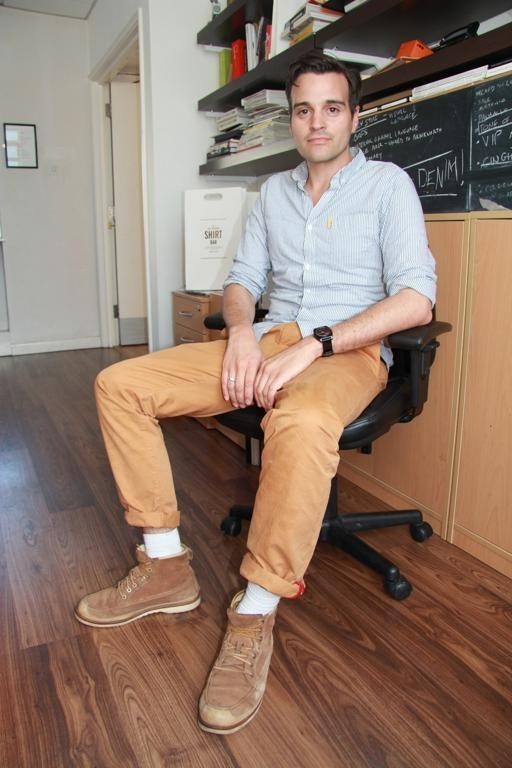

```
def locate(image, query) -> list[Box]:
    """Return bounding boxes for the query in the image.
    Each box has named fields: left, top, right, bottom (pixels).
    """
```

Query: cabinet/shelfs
left=196, top=0, right=511, bottom=177
left=335, top=217, right=512, bottom=580
left=169, top=290, right=216, bottom=429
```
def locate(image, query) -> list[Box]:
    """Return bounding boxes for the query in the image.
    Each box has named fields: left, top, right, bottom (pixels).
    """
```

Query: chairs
left=202, top=296, right=452, bottom=604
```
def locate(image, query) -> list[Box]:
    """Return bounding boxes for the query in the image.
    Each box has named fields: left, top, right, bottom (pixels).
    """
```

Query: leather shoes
left=197, top=588, right=277, bottom=735
left=73, top=544, right=200, bottom=628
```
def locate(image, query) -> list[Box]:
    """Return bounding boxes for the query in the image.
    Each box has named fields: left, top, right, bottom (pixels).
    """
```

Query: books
left=323, top=46, right=391, bottom=72
left=202, top=1, right=294, bottom=164
left=295, top=1, right=397, bottom=43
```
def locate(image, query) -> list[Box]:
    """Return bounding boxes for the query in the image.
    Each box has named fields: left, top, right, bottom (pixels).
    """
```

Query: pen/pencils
left=326, top=214, right=332, bottom=229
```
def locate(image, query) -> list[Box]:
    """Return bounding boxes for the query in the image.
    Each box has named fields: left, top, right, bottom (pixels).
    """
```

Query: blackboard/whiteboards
left=347, top=70, right=512, bottom=220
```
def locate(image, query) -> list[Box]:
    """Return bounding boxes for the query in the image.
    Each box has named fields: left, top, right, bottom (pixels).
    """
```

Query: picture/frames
left=3, top=123, right=39, bottom=169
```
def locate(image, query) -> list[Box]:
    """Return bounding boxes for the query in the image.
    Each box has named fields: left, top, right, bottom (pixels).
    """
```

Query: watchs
left=311, top=324, right=335, bottom=359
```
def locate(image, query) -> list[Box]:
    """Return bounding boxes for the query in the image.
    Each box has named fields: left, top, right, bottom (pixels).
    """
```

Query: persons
left=71, top=50, right=440, bottom=739
